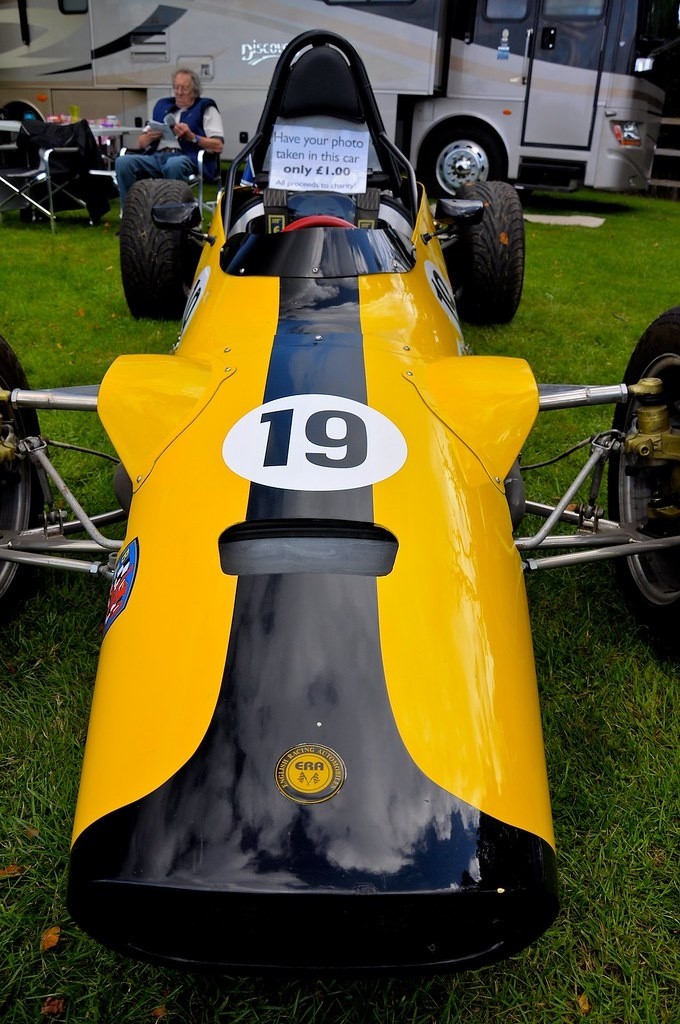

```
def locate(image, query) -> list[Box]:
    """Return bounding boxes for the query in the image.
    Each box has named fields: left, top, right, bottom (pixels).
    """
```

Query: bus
left=1, top=0, right=679, bottom=211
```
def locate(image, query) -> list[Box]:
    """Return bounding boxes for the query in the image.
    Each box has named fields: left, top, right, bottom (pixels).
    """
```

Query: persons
left=115, top=68, right=224, bottom=207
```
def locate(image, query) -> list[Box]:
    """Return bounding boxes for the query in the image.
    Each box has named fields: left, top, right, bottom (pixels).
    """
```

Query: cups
left=70, top=105, right=80, bottom=124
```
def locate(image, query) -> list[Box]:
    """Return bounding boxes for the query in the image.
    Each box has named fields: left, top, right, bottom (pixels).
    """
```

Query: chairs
left=0, top=117, right=100, bottom=236
left=271, top=47, right=368, bottom=194
left=1, top=98, right=45, bottom=122
left=118, top=96, right=221, bottom=221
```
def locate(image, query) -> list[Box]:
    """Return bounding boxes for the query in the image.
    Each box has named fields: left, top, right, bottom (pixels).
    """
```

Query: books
left=147, top=113, right=177, bottom=140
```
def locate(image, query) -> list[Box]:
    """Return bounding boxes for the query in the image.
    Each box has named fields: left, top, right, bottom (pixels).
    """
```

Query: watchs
left=192, top=134, right=199, bottom=145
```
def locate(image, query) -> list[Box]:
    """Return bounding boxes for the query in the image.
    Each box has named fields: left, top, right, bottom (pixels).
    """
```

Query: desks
left=0, top=121, right=141, bottom=222
